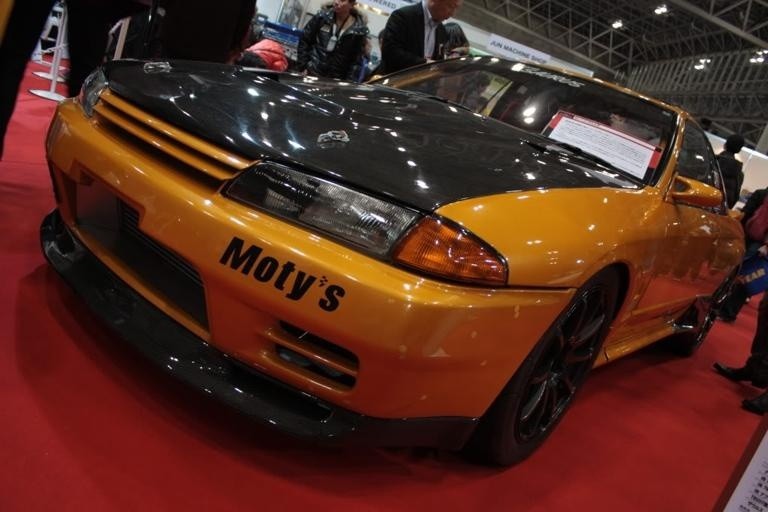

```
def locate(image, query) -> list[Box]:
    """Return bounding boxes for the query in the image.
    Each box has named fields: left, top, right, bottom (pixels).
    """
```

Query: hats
left=37, top=51, right=751, bottom=470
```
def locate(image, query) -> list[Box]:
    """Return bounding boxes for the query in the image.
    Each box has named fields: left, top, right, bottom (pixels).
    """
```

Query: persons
left=713, top=134, right=767, bottom=416
left=296, top=0, right=492, bottom=115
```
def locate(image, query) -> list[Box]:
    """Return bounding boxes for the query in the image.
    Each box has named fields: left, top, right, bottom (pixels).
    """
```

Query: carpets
left=426, top=21, right=438, bottom=58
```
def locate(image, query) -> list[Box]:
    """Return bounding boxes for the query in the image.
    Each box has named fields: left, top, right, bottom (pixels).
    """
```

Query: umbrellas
left=742, top=390, right=768, bottom=414
left=713, top=354, right=768, bottom=389
